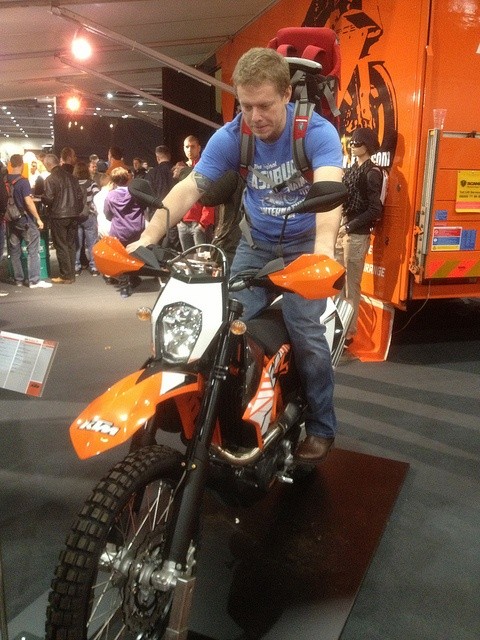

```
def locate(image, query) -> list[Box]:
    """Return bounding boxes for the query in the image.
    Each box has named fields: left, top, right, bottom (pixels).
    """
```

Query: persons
left=3, top=154, right=52, bottom=288
left=171, top=160, right=244, bottom=270
left=88, top=162, right=98, bottom=180
left=339, top=128, right=381, bottom=354
left=175, top=134, right=216, bottom=254
left=60, top=149, right=78, bottom=176
left=72, top=161, right=101, bottom=277
left=102, top=147, right=133, bottom=186
left=124, top=47, right=344, bottom=466
left=0, top=160, right=9, bottom=295
left=89, top=153, right=100, bottom=163
left=143, top=143, right=179, bottom=282
left=98, top=162, right=109, bottom=173
left=140, top=160, right=150, bottom=174
left=41, top=154, right=84, bottom=284
left=94, top=166, right=131, bottom=238
left=103, top=167, right=144, bottom=295
left=132, top=158, right=146, bottom=178
left=28, top=160, right=42, bottom=189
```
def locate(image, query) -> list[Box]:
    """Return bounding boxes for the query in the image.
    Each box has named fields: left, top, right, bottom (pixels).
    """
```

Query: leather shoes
left=51, top=276, right=70, bottom=283
left=294, top=436, right=331, bottom=465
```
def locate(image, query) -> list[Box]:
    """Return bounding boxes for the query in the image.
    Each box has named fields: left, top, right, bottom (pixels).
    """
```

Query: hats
left=353, top=128, right=381, bottom=155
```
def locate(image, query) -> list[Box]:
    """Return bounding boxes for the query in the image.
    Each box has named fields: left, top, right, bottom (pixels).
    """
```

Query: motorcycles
left=44, top=181, right=354, bottom=640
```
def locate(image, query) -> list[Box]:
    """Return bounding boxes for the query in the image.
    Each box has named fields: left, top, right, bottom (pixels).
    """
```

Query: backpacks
left=238, top=27, right=342, bottom=189
left=3, top=174, right=24, bottom=224
left=80, top=184, right=91, bottom=222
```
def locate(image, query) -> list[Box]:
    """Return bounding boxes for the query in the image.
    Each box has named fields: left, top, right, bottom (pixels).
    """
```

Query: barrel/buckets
left=6, top=201, right=52, bottom=279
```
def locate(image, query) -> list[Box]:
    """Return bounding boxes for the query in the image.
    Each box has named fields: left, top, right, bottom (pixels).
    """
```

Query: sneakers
left=29, top=281, right=52, bottom=288
left=88, top=266, right=99, bottom=276
left=75, top=267, right=84, bottom=276
left=121, top=289, right=129, bottom=296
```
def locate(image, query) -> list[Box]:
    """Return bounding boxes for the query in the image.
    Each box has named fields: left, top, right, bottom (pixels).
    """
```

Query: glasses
left=350, top=141, right=367, bottom=146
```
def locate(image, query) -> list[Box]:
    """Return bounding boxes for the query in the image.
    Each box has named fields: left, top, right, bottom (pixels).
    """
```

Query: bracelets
left=344, top=223, right=350, bottom=233
